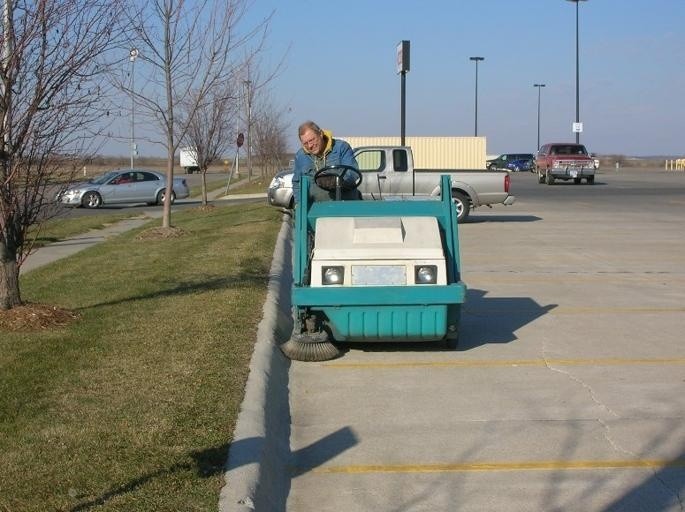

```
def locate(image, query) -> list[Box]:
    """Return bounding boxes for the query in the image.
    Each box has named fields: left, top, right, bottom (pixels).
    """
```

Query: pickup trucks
left=535, top=142, right=596, bottom=186
left=267, top=146, right=515, bottom=224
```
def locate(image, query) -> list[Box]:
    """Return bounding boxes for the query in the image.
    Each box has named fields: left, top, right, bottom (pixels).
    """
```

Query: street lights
left=470, top=56, right=484, bottom=137
left=533, top=84, right=545, bottom=153
left=243, top=80, right=253, bottom=175
left=129, top=48, right=139, bottom=168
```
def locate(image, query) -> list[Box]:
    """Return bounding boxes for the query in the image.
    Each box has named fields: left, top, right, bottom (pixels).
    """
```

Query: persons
left=111, top=173, right=137, bottom=184
left=292, top=121, right=362, bottom=209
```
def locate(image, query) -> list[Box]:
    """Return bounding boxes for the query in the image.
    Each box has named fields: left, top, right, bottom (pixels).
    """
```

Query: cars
left=589, top=154, right=600, bottom=169
left=56, top=168, right=189, bottom=209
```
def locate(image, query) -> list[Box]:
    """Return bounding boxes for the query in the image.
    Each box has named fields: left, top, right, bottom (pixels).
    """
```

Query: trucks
left=180, top=146, right=201, bottom=174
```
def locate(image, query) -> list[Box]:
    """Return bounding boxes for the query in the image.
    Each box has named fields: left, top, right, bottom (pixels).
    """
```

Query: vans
left=486, top=154, right=535, bottom=173
